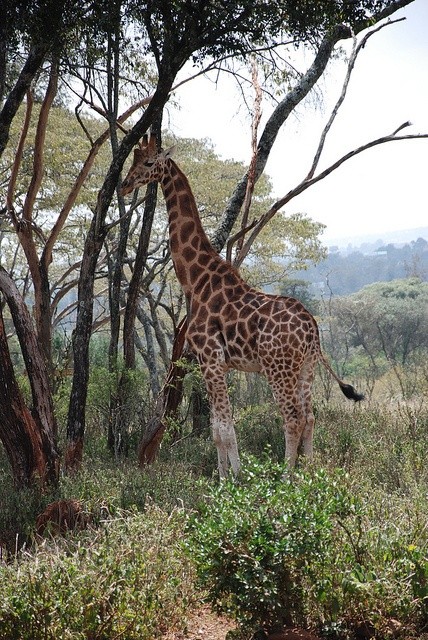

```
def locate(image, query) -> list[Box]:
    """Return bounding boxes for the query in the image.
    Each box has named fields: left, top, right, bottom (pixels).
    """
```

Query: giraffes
left=120, top=131, right=366, bottom=485
